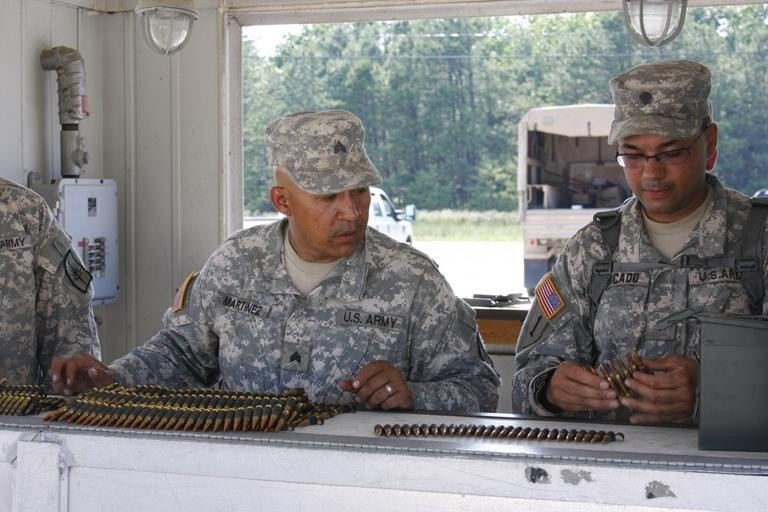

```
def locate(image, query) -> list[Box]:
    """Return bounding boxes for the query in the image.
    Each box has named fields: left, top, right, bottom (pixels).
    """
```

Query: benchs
left=527, top=156, right=565, bottom=209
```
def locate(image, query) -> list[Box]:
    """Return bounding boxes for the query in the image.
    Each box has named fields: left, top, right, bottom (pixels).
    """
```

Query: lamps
left=135, top=0, right=199, bottom=55
left=623, top=1, right=687, bottom=47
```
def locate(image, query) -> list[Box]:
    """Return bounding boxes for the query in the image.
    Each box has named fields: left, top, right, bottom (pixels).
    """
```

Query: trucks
left=517, top=103, right=631, bottom=298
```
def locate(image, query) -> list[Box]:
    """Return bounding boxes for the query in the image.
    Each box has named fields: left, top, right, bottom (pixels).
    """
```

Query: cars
left=366, top=186, right=416, bottom=245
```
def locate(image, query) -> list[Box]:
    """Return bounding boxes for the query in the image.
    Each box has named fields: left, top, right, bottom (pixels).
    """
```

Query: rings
left=384, top=383, right=394, bottom=397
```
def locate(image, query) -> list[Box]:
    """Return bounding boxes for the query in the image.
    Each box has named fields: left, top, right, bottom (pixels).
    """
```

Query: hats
left=264, top=110, right=383, bottom=195
left=607, top=59, right=712, bottom=146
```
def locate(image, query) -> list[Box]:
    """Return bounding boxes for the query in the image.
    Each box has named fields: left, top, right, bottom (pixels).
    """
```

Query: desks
left=0, top=403, right=768, bottom=511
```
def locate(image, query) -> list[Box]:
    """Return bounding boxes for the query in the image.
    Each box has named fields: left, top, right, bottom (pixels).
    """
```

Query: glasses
left=615, top=126, right=707, bottom=169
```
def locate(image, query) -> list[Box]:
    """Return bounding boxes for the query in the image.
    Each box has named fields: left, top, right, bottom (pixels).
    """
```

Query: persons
left=0, top=177, right=98, bottom=415
left=48, top=106, right=499, bottom=413
left=509, top=60, right=766, bottom=428
